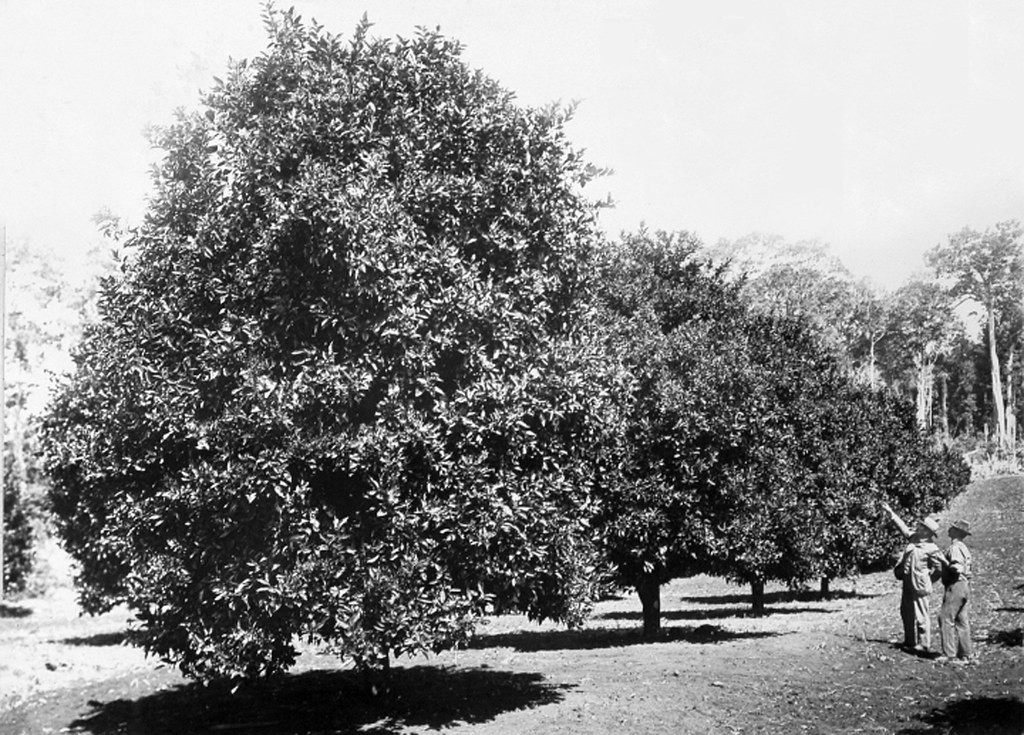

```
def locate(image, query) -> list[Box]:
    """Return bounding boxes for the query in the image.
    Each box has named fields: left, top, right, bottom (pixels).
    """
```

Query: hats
left=950, top=519, right=972, bottom=536
left=916, top=517, right=939, bottom=538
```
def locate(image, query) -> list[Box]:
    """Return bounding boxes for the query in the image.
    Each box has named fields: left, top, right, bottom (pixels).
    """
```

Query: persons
left=931, top=519, right=972, bottom=661
left=879, top=502, right=942, bottom=652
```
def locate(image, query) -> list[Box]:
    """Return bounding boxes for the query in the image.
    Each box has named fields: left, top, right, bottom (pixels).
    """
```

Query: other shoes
left=935, top=654, right=954, bottom=663
